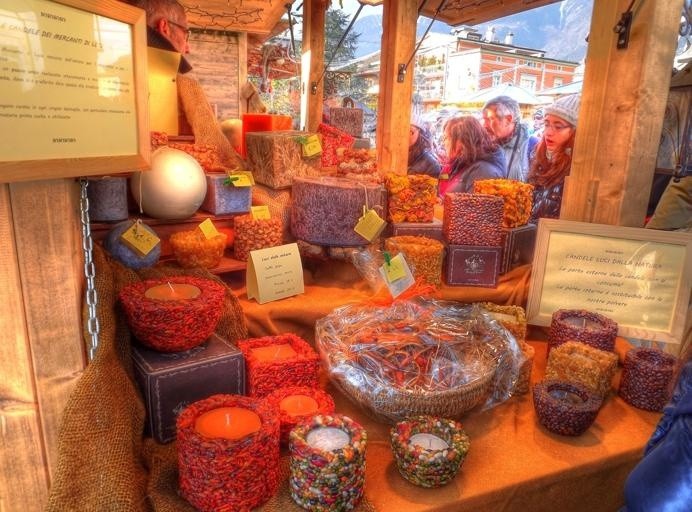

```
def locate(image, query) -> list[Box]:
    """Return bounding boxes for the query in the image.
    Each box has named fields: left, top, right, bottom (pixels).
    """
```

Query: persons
left=409, top=95, right=582, bottom=225
left=128, top=0, right=247, bottom=167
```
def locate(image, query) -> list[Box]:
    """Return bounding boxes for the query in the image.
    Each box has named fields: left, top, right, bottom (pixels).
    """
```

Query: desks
left=317, top=327, right=669, bottom=512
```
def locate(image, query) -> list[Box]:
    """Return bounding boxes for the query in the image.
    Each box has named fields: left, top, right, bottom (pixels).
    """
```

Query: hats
left=543, top=92, right=582, bottom=126
left=411, top=112, right=427, bottom=133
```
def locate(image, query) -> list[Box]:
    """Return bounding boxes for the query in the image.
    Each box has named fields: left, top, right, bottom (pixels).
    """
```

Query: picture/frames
left=525, top=219, right=692, bottom=346
left=1, top=1, right=152, bottom=183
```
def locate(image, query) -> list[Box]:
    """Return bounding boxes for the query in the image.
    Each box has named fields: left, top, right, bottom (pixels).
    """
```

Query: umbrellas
left=537, top=78, right=585, bottom=96
left=469, top=82, right=541, bottom=107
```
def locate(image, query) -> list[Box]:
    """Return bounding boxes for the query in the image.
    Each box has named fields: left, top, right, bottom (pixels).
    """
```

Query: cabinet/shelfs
left=87, top=134, right=534, bottom=359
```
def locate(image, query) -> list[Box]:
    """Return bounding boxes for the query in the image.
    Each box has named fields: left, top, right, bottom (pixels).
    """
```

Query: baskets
left=328, top=97, right=364, bottom=138
left=314, top=305, right=511, bottom=421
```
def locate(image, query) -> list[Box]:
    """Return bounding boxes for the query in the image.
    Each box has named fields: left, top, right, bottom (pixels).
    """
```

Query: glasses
left=166, top=20, right=191, bottom=42
left=543, top=119, right=571, bottom=131
